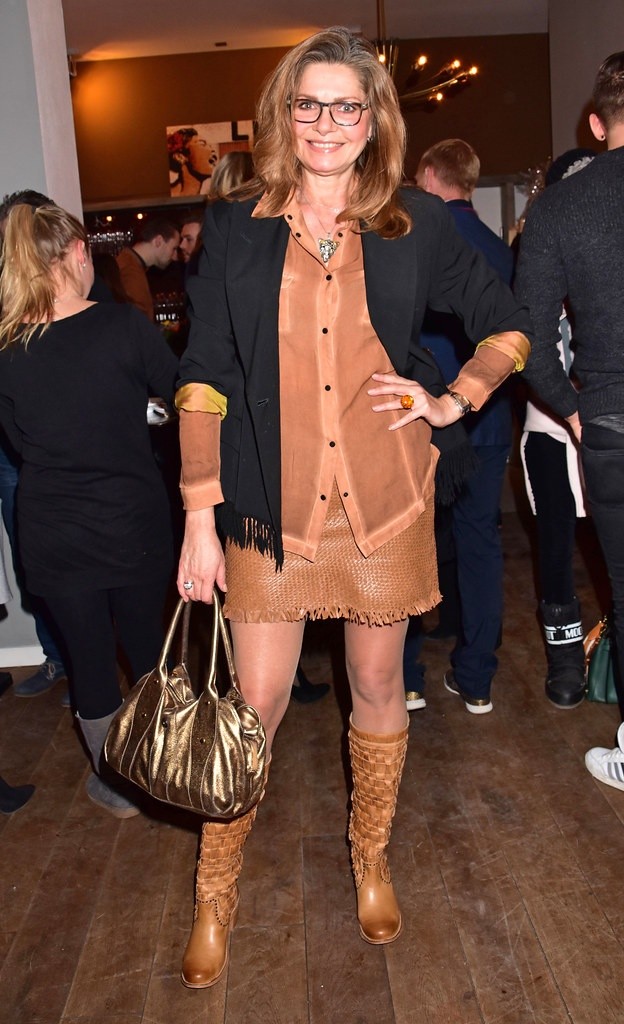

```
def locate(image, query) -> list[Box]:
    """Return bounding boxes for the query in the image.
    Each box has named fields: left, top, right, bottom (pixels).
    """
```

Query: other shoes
left=405, top=686, right=427, bottom=710
left=421, top=621, right=463, bottom=640
left=443, top=668, right=493, bottom=713
left=0, top=672, right=12, bottom=696
left=61, top=687, right=71, bottom=708
left=13, top=658, right=67, bottom=698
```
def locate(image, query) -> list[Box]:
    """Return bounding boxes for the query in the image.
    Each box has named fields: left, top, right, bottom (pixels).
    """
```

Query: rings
left=184, top=581, right=192, bottom=590
left=400, top=395, right=414, bottom=409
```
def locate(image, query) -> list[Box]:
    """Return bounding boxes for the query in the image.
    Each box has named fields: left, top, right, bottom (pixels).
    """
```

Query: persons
left=208, top=152, right=254, bottom=196
left=113, top=213, right=202, bottom=324
left=402, top=53, right=624, bottom=792
left=172, top=26, right=528, bottom=990
left=0, top=189, right=196, bottom=819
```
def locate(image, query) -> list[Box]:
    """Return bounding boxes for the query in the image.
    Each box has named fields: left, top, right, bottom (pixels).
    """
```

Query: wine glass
left=86, top=224, right=133, bottom=257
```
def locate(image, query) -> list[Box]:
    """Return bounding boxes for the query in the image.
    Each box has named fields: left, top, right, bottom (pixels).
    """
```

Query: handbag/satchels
left=103, top=589, right=270, bottom=817
left=576, top=620, right=618, bottom=704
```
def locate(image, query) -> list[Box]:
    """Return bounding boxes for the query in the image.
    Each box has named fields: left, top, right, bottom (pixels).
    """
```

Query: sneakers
left=585, top=746, right=624, bottom=791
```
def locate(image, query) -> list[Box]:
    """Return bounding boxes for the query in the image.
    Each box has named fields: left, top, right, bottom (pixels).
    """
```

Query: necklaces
left=52, top=295, right=72, bottom=303
left=299, top=188, right=348, bottom=262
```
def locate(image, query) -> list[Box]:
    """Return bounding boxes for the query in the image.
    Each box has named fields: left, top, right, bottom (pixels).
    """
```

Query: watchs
left=445, top=390, right=470, bottom=416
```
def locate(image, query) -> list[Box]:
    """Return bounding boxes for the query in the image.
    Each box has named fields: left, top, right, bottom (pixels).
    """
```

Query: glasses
left=286, top=98, right=371, bottom=127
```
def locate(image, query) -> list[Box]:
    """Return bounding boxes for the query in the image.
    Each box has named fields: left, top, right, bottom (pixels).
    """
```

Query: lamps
left=374, top=0, right=478, bottom=104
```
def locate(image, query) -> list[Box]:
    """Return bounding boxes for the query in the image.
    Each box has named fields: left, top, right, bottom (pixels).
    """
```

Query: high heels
left=0, top=777, right=35, bottom=815
left=287, top=667, right=332, bottom=703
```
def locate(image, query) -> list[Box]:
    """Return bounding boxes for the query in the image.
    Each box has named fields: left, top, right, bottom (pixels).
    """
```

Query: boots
left=536, top=596, right=585, bottom=709
left=77, top=700, right=140, bottom=818
left=180, top=803, right=258, bottom=988
left=349, top=711, right=411, bottom=945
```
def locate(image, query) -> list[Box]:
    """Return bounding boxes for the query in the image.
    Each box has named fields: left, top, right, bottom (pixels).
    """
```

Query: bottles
left=154, top=289, right=187, bottom=326
